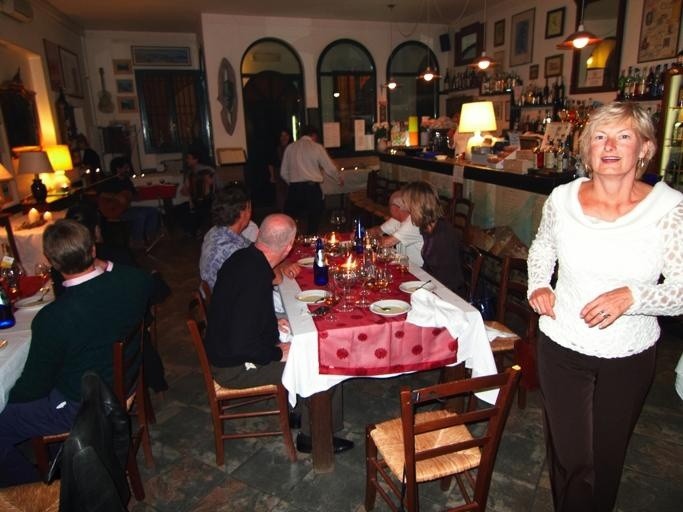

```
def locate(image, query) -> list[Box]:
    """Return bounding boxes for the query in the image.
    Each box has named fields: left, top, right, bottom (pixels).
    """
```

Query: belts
left=290, top=182, right=320, bottom=186
left=211, top=358, right=255, bottom=367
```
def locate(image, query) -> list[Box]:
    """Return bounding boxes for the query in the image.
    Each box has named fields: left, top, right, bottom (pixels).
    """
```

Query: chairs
left=31, top=315, right=153, bottom=501
left=97, top=190, right=132, bottom=248
left=466, top=253, right=536, bottom=413
left=189, top=170, right=215, bottom=216
left=450, top=198, right=474, bottom=228
left=364, top=364, right=521, bottom=512
left=187, top=319, right=298, bottom=465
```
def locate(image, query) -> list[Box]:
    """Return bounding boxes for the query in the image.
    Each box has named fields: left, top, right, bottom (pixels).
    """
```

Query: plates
left=399, top=281, right=435, bottom=294
left=15, top=295, right=54, bottom=311
left=294, top=289, right=330, bottom=304
left=297, top=258, right=327, bottom=267
left=369, top=299, right=411, bottom=317
left=435, top=155, right=447, bottom=160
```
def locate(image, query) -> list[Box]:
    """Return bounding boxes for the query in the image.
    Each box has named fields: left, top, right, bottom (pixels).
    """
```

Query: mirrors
left=568, top=1, right=626, bottom=94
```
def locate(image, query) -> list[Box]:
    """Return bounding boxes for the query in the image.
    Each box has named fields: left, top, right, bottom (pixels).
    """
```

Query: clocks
left=544, top=6, right=564, bottom=40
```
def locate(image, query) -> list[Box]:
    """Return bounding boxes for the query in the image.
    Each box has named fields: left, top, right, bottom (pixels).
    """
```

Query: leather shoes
left=297, top=432, right=354, bottom=453
left=290, top=412, right=302, bottom=427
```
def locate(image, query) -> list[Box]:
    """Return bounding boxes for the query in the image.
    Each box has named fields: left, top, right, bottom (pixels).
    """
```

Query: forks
left=35, top=288, right=51, bottom=304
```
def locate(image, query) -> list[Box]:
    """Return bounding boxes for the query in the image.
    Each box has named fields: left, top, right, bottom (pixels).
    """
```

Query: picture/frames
left=507, top=7, right=535, bottom=67
left=42, top=38, right=62, bottom=92
left=130, top=44, right=192, bottom=67
left=111, top=58, right=133, bottom=75
left=60, top=38, right=84, bottom=99
left=115, top=78, right=134, bottom=93
left=492, top=18, right=505, bottom=47
left=116, top=95, right=137, bottom=114
left=543, top=54, right=563, bottom=78
left=636, top=0, right=681, bottom=63
left=528, top=64, right=538, bottom=80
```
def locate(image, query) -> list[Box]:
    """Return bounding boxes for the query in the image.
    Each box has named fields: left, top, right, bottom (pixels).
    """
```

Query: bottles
left=647, top=64, right=655, bottom=86
left=632, top=68, right=641, bottom=96
left=534, top=139, right=544, bottom=169
left=660, top=64, right=667, bottom=90
left=557, top=75, right=564, bottom=104
left=354, top=217, right=362, bottom=253
left=640, top=67, right=647, bottom=96
left=652, top=65, right=661, bottom=87
left=523, top=109, right=551, bottom=133
left=564, top=140, right=570, bottom=155
left=557, top=142, right=569, bottom=172
left=544, top=139, right=555, bottom=169
left=542, top=77, right=549, bottom=104
left=624, top=66, right=634, bottom=99
left=313, top=238, right=328, bottom=285
left=444, top=69, right=449, bottom=92
left=617, top=70, right=625, bottom=99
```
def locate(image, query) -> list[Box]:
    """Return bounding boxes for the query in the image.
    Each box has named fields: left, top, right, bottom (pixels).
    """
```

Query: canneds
left=0, top=285, right=9, bottom=304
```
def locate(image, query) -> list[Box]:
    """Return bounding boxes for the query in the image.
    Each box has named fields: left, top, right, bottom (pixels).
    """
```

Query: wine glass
left=377, top=245, right=391, bottom=275
left=334, top=267, right=353, bottom=312
left=380, top=276, right=391, bottom=293
left=355, top=266, right=370, bottom=308
left=325, top=285, right=339, bottom=321
left=330, top=212, right=344, bottom=234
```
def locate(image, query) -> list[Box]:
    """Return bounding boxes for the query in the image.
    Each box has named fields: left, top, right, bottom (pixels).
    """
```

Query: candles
left=338, top=254, right=359, bottom=289
left=326, top=232, right=339, bottom=254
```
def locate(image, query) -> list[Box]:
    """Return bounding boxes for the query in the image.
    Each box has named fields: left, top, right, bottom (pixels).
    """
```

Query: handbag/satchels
left=514, top=308, right=540, bottom=390
left=463, top=295, right=494, bottom=320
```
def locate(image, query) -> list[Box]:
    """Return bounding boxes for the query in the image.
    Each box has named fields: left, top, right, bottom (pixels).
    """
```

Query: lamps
left=16, top=150, right=55, bottom=202
left=458, top=101, right=497, bottom=165
left=467, top=0, right=498, bottom=70
left=415, top=1, right=442, bottom=82
left=44, top=144, right=73, bottom=191
left=555, top=1, right=602, bottom=50
left=382, top=5, right=401, bottom=89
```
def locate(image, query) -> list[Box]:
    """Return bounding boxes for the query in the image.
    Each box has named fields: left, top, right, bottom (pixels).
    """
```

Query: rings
left=600, top=310, right=607, bottom=318
left=533, top=307, right=538, bottom=312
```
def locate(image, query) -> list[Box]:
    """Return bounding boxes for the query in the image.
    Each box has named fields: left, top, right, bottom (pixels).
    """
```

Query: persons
left=279, top=124, right=344, bottom=234
left=174, top=148, right=216, bottom=246
left=205, top=213, right=357, bottom=456
left=97, top=156, right=138, bottom=266
left=365, top=190, right=425, bottom=268
left=77, top=133, right=103, bottom=174
left=198, top=180, right=302, bottom=296
left=401, top=179, right=466, bottom=320
left=267, top=127, right=291, bottom=213
left=525, top=102, right=683, bottom=511
left=1, top=217, right=174, bottom=491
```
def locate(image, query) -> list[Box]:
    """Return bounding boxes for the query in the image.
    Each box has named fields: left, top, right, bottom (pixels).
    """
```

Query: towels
left=406, top=288, right=470, bottom=339
left=273, top=290, right=285, bottom=313
left=278, top=325, right=290, bottom=343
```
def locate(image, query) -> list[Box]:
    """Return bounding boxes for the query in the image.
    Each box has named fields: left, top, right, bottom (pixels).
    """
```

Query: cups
left=304, top=235, right=311, bottom=248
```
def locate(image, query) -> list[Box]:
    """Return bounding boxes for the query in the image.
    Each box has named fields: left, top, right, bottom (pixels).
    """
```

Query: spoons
left=416, top=280, right=431, bottom=290
left=373, top=304, right=391, bottom=311
